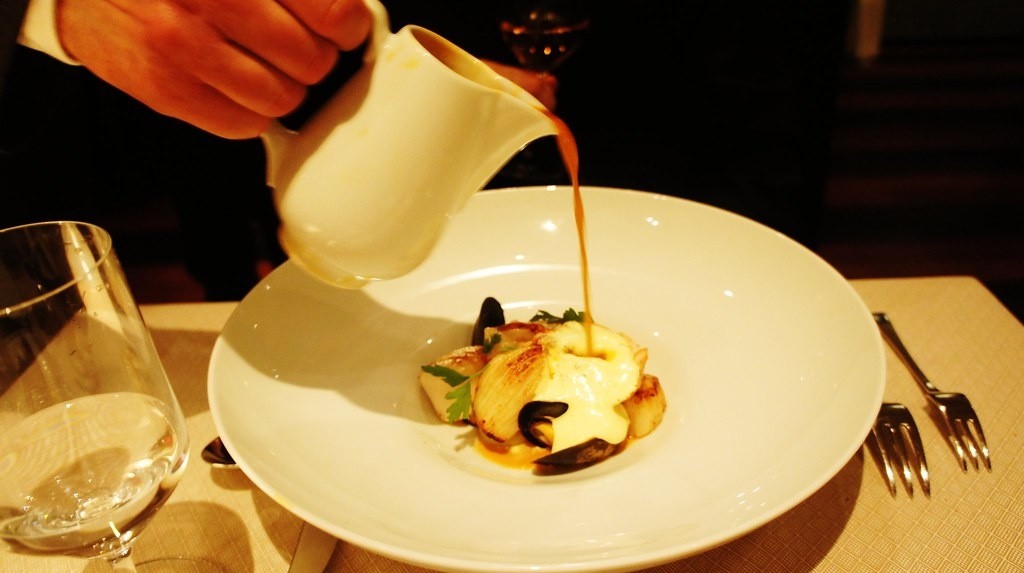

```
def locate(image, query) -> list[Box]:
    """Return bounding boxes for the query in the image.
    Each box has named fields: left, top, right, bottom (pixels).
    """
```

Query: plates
left=206, top=185, right=887, bottom=573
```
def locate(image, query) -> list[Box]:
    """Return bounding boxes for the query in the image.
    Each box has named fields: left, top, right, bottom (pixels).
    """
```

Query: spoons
left=200, top=436, right=240, bottom=470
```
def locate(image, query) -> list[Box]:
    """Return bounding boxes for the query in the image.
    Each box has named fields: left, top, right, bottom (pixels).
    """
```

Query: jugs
left=262, top=0, right=561, bottom=284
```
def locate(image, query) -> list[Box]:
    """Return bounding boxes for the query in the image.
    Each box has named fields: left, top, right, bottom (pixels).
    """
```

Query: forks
left=872, top=402, right=931, bottom=500
left=872, top=311, right=992, bottom=474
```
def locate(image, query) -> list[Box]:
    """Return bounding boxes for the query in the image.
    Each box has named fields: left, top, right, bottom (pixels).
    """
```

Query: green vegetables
left=419, top=363, right=489, bottom=423
left=530, top=308, right=593, bottom=324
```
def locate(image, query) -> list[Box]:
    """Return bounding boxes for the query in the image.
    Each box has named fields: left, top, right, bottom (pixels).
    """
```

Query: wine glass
left=0, top=220, right=223, bottom=573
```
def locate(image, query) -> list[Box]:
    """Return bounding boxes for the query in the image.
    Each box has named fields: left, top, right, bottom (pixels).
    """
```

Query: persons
left=1, top=0, right=379, bottom=338
